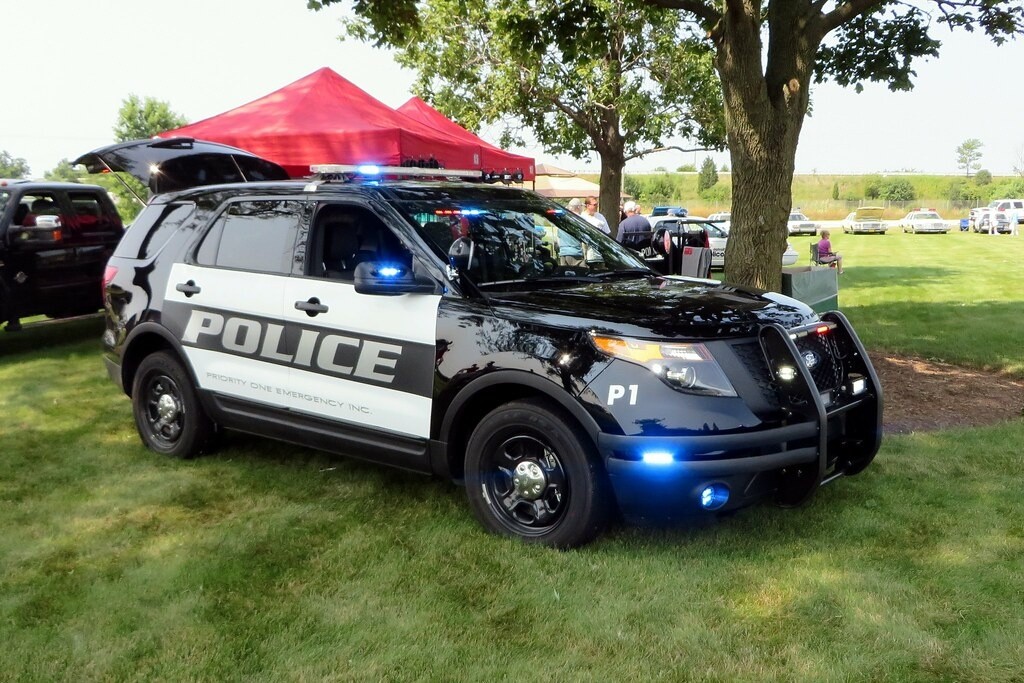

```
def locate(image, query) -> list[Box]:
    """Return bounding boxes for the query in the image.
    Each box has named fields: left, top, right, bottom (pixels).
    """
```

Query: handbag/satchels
left=992, top=220, right=999, bottom=226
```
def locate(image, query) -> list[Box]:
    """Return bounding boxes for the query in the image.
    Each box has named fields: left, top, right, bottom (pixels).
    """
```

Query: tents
left=393, top=95, right=536, bottom=195
left=148, top=66, right=482, bottom=185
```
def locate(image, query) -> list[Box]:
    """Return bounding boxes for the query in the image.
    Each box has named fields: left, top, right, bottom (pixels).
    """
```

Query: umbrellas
left=506, top=163, right=634, bottom=258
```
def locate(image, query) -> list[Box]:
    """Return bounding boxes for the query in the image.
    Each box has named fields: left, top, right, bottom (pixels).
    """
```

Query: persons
left=818, top=229, right=845, bottom=274
left=582, top=196, right=611, bottom=273
left=615, top=200, right=652, bottom=252
left=557, top=198, right=587, bottom=269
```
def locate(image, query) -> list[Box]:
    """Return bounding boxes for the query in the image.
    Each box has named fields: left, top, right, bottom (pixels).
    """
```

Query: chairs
left=31, top=199, right=56, bottom=215
left=810, top=242, right=837, bottom=268
left=424, top=221, right=454, bottom=256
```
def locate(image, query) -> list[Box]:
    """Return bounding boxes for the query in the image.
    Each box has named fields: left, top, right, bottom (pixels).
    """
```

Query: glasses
left=588, top=204, right=597, bottom=206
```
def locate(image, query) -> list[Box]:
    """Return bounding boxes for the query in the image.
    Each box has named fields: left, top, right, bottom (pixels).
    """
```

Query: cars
left=843, top=207, right=888, bottom=235
left=641, top=211, right=743, bottom=270
left=70, top=135, right=888, bottom=546
left=968, top=198, right=1024, bottom=234
left=705, top=211, right=799, bottom=268
left=785, top=212, right=819, bottom=236
left=0, top=177, right=127, bottom=330
left=900, top=208, right=951, bottom=234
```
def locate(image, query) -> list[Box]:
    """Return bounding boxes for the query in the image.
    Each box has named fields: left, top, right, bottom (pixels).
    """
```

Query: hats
left=624, top=201, right=636, bottom=211
left=568, top=198, right=586, bottom=206
